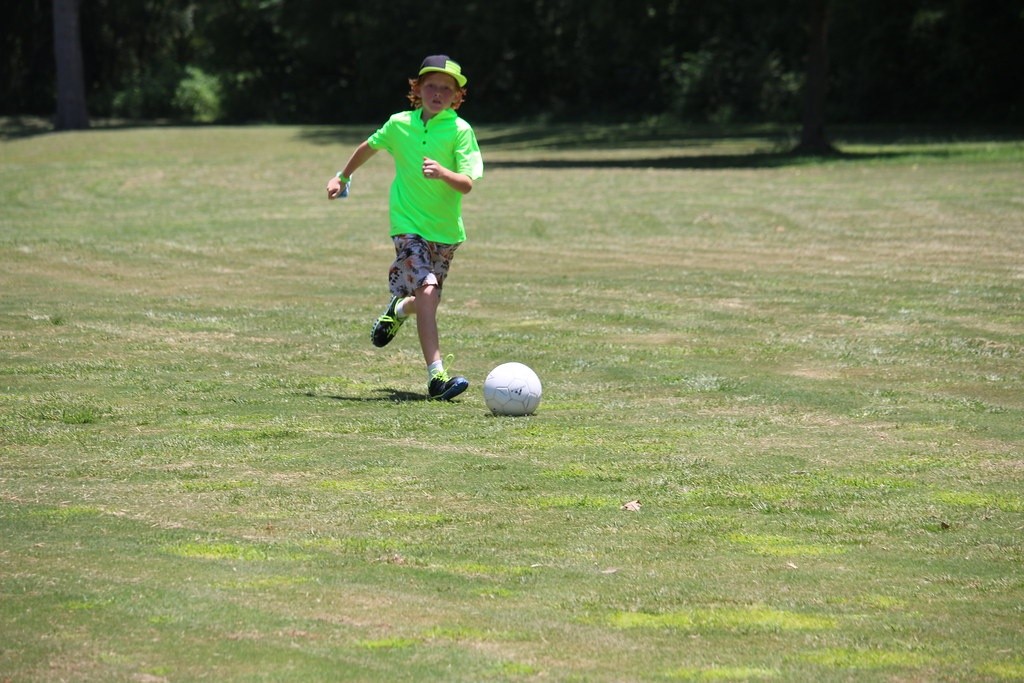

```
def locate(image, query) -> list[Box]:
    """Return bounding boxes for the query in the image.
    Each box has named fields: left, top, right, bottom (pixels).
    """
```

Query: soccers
left=483, top=361, right=543, bottom=416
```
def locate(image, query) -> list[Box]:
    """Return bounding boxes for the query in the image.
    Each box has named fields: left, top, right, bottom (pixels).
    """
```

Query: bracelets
left=337, top=171, right=350, bottom=183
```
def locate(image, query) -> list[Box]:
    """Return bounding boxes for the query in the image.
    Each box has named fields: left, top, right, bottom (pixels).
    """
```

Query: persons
left=326, top=55, right=483, bottom=400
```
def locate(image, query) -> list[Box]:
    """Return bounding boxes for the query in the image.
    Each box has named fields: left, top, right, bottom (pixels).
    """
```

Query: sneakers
left=371, top=293, right=409, bottom=347
left=427, top=354, right=468, bottom=400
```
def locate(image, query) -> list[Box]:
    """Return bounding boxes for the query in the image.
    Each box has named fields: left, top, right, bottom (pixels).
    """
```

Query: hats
left=418, top=55, right=467, bottom=87
